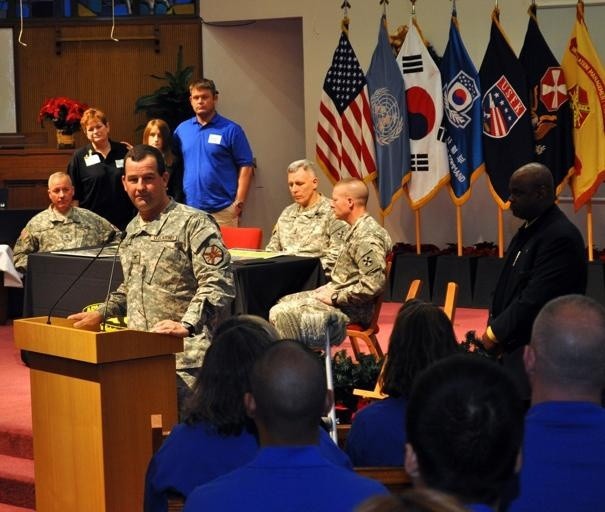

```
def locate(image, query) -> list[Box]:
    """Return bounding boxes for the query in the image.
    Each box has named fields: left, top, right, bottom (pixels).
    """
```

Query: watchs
left=182, top=320, right=197, bottom=336
left=331, top=291, right=340, bottom=304
left=233, top=199, right=246, bottom=212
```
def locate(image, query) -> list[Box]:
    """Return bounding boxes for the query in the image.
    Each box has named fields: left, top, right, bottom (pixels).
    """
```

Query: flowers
left=38, top=97, right=91, bottom=128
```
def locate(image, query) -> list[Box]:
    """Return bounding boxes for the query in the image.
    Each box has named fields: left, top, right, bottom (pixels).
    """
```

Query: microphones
left=100, top=231, right=127, bottom=332
left=46, top=229, right=116, bottom=325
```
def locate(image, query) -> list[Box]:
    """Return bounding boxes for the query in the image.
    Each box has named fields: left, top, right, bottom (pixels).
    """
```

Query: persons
left=264, top=159, right=349, bottom=281
left=143, top=119, right=183, bottom=203
left=270, top=179, right=394, bottom=339
left=343, top=301, right=460, bottom=468
left=12, top=171, right=123, bottom=273
left=121, top=78, right=254, bottom=226
left=480, top=165, right=587, bottom=356
left=405, top=353, right=527, bottom=512
left=68, top=109, right=139, bottom=231
left=507, top=294, right=605, bottom=512
left=145, top=315, right=353, bottom=511
left=68, top=144, right=236, bottom=415
left=179, top=338, right=390, bottom=512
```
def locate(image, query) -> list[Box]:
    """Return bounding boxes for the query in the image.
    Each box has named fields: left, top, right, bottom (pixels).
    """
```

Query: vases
left=56, top=130, right=78, bottom=149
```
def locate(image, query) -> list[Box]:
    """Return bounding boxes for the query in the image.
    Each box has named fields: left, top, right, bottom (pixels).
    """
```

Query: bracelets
left=486, top=326, right=501, bottom=342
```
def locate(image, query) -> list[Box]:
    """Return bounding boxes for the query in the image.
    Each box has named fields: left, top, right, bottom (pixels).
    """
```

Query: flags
left=442, top=8, right=486, bottom=205
left=315, top=20, right=378, bottom=189
left=479, top=8, right=539, bottom=211
left=564, top=1, right=605, bottom=212
left=397, top=13, right=452, bottom=210
left=366, top=14, right=412, bottom=216
left=518, top=0, right=582, bottom=204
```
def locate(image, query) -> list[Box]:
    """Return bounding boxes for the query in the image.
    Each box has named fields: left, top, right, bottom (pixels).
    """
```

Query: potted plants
left=135, top=62, right=206, bottom=204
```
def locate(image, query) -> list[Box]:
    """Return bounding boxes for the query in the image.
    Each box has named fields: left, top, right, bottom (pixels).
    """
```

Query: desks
left=27, top=241, right=322, bottom=329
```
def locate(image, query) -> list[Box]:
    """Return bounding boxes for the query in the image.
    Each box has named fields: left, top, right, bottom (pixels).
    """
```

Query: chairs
left=217, top=224, right=264, bottom=254
left=344, top=258, right=393, bottom=365
left=431, top=254, right=473, bottom=308
left=163, top=461, right=413, bottom=512
left=473, top=254, right=509, bottom=309
left=585, top=261, right=605, bottom=305
left=391, top=251, right=433, bottom=305
